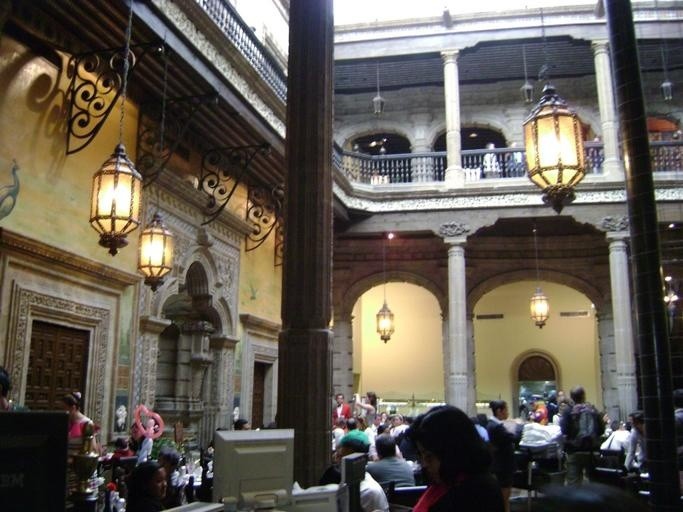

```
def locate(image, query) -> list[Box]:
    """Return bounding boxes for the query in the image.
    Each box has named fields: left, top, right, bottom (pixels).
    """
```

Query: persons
left=0, top=362, right=683, bottom=512
left=483, top=143, right=502, bottom=178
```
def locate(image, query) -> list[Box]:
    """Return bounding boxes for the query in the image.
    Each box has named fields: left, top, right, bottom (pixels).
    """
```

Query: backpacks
left=569, top=402, right=599, bottom=450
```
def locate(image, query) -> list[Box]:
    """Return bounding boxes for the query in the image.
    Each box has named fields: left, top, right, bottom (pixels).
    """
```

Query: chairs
left=183, top=436, right=651, bottom=512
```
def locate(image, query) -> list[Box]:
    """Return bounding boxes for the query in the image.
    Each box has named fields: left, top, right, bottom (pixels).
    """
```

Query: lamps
left=517, top=42, right=537, bottom=104
left=371, top=62, right=387, bottom=118
left=135, top=53, right=178, bottom=292
left=520, top=5, right=589, bottom=217
left=530, top=226, right=551, bottom=330
left=659, top=44, right=675, bottom=103
left=661, top=275, right=678, bottom=310
left=90, top=0, right=146, bottom=258
left=375, top=232, right=397, bottom=345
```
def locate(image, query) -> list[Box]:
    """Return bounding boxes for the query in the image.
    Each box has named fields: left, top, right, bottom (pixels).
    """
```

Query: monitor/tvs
left=212, top=428, right=296, bottom=511
left=517, top=442, right=560, bottom=474
